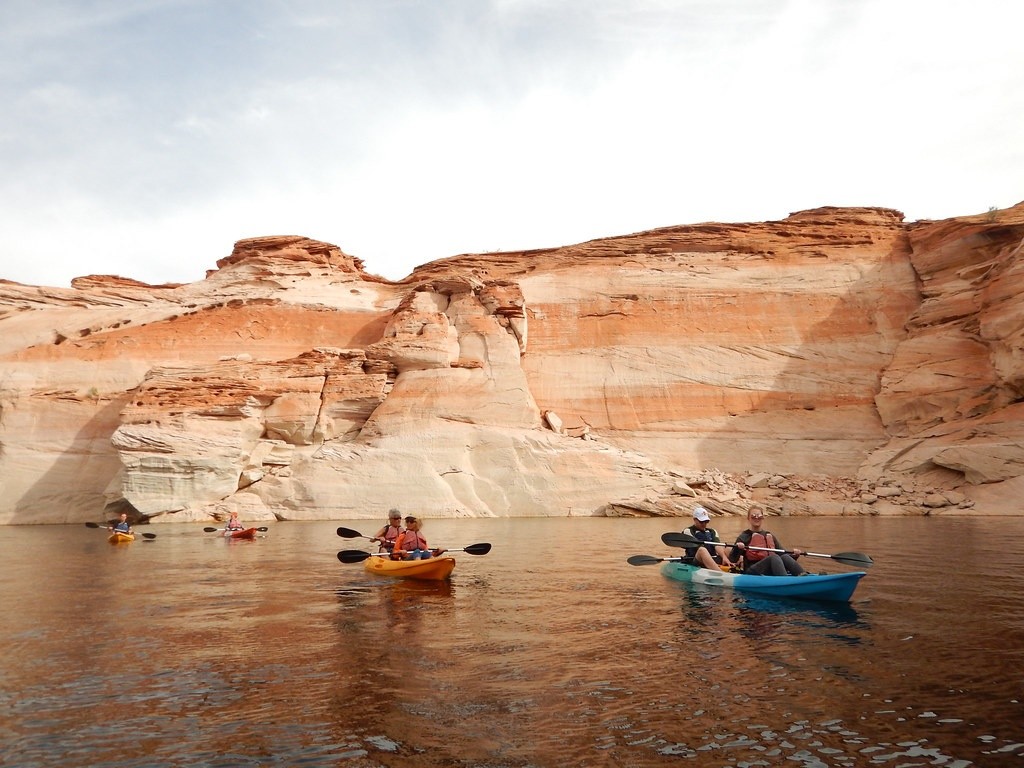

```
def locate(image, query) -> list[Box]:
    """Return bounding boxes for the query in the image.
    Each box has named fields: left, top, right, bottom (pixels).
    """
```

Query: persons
left=108, top=512, right=133, bottom=535
left=370, top=509, right=404, bottom=559
left=682, top=508, right=743, bottom=573
left=729, top=505, right=809, bottom=576
left=225, top=513, right=244, bottom=531
left=393, top=513, right=444, bottom=560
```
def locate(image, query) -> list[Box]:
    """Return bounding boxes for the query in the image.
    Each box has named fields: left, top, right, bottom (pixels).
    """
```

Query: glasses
left=697, top=519, right=709, bottom=523
left=391, top=517, right=401, bottom=520
left=750, top=514, right=763, bottom=519
left=406, top=519, right=416, bottom=523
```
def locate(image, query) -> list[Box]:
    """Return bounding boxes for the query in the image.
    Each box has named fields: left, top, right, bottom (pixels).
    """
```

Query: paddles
left=337, top=543, right=491, bottom=564
left=627, top=554, right=718, bottom=566
left=661, top=532, right=875, bottom=568
left=85, top=522, right=157, bottom=539
left=204, top=527, right=268, bottom=532
left=336, top=527, right=394, bottom=545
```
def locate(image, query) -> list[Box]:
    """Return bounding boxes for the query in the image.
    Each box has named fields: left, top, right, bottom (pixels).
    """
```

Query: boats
left=363, top=556, right=456, bottom=580
left=219, top=527, right=257, bottom=538
left=108, top=532, right=136, bottom=542
left=661, top=555, right=867, bottom=601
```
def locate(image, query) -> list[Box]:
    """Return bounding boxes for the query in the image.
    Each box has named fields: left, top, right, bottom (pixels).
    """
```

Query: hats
left=693, top=508, right=710, bottom=522
left=405, top=513, right=418, bottom=519
